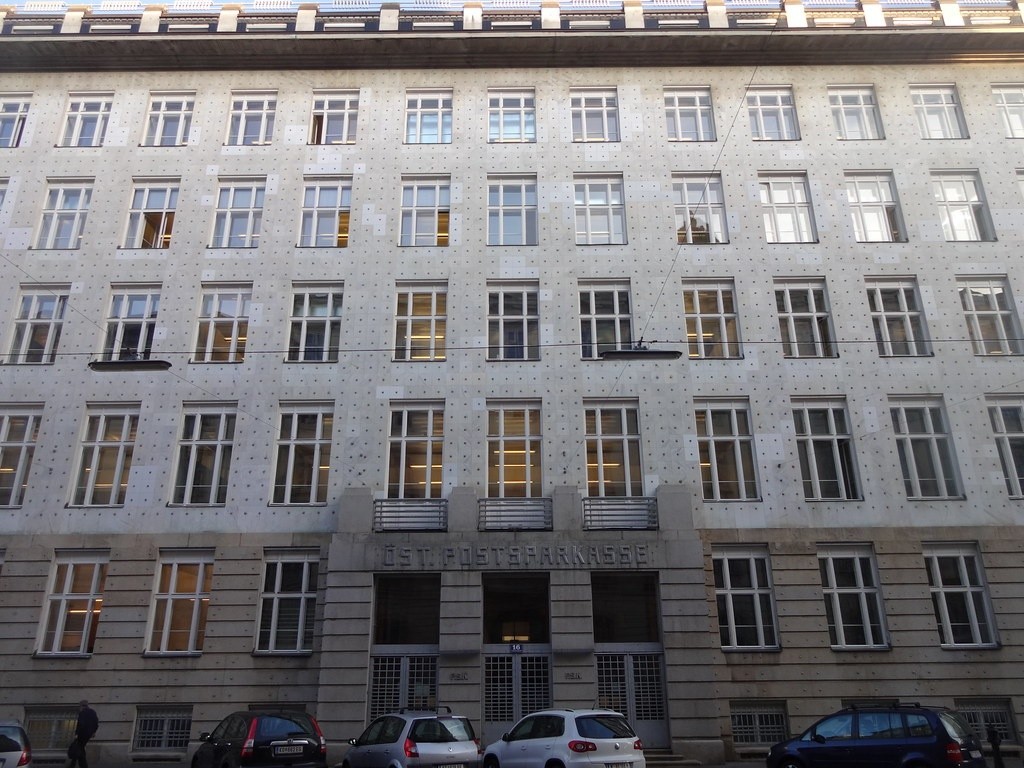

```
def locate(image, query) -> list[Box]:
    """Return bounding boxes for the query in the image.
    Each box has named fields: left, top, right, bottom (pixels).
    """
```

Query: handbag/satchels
left=67, top=737, right=80, bottom=759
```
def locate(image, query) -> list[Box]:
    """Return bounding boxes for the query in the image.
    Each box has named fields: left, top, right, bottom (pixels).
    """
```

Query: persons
left=67, top=700, right=99, bottom=768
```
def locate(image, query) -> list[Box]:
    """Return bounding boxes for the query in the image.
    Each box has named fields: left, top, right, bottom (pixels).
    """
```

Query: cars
left=342, top=706, right=483, bottom=768
left=190, top=710, right=327, bottom=768
left=482, top=707, right=646, bottom=768
left=771, top=699, right=986, bottom=768
left=0, top=722, right=33, bottom=768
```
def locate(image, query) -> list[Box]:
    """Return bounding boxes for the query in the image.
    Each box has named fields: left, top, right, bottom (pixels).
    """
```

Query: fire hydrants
left=985, top=722, right=1006, bottom=768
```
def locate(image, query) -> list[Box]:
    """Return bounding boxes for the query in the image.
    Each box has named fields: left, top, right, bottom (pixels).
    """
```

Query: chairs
left=861, top=720, right=878, bottom=737
left=877, top=715, right=888, bottom=735
left=422, top=724, right=437, bottom=737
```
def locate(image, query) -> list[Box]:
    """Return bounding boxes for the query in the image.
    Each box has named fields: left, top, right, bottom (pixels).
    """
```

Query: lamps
left=88, top=360, right=172, bottom=371
left=598, top=350, right=683, bottom=361
